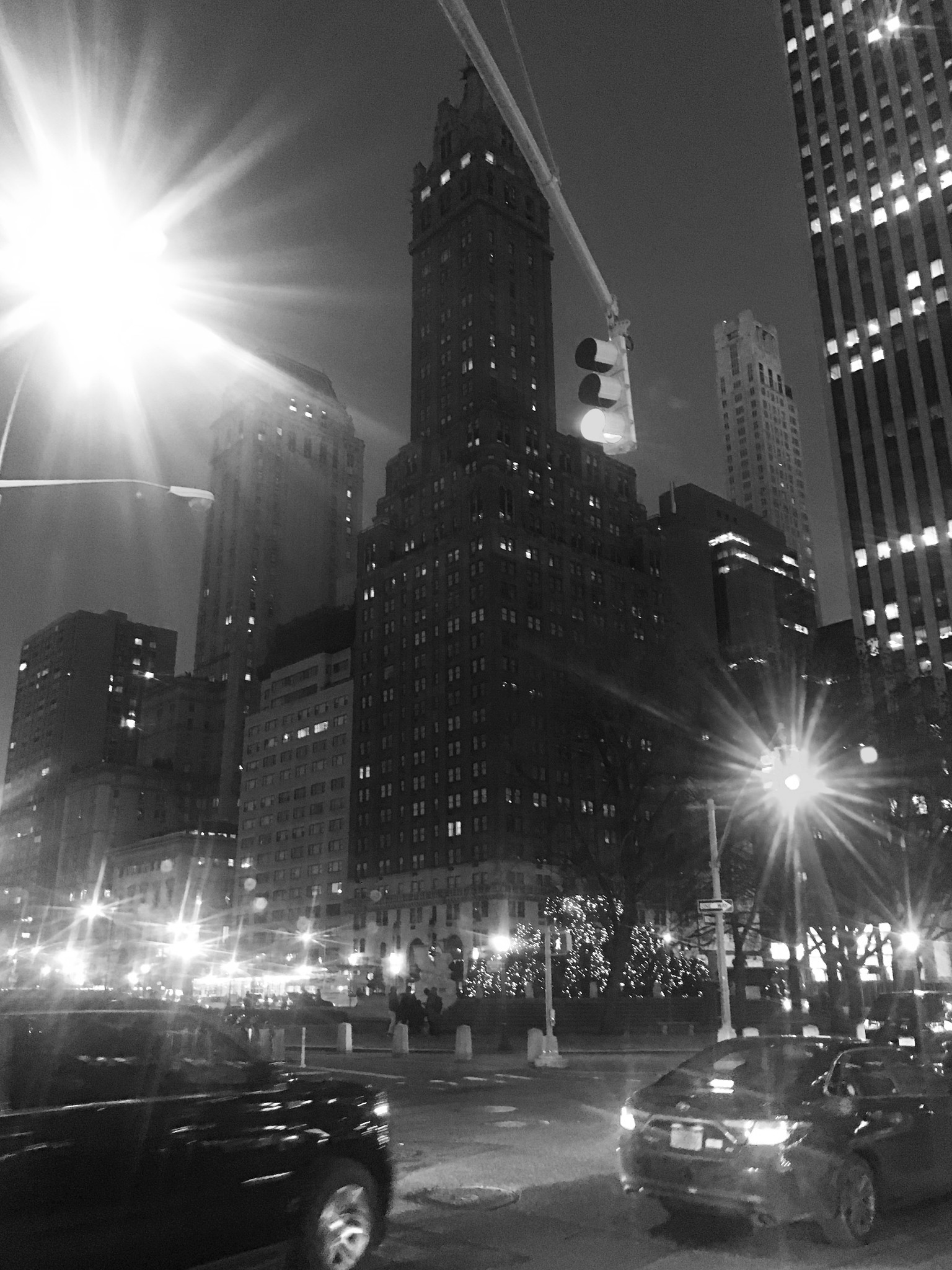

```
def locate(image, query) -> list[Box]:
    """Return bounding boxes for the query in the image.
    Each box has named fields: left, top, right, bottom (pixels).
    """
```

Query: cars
left=618, top=1035, right=952, bottom=1247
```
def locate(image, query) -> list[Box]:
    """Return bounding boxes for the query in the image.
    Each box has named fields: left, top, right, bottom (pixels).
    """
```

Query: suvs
left=0, top=991, right=399, bottom=1270
left=864, top=990, right=952, bottom=1070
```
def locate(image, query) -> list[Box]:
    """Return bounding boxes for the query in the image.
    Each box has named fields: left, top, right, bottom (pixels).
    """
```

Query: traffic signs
left=574, top=337, right=638, bottom=456
left=697, top=898, right=734, bottom=914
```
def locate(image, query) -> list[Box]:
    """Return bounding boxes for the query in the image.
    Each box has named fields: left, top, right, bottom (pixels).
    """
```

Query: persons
left=312, top=988, right=322, bottom=1007
left=386, top=986, right=442, bottom=1037
